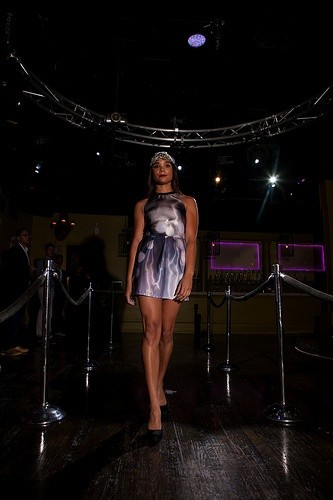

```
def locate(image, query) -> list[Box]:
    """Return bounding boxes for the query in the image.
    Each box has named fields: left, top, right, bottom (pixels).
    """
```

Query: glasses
left=21, top=234, right=31, bottom=236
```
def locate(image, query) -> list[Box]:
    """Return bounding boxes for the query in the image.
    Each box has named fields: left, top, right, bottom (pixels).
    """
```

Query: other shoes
left=0, top=346, right=29, bottom=356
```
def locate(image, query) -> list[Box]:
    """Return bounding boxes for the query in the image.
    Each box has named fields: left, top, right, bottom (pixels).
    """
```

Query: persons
left=35, top=243, right=66, bottom=344
left=126, top=151, right=199, bottom=440
left=0, top=228, right=32, bottom=355
